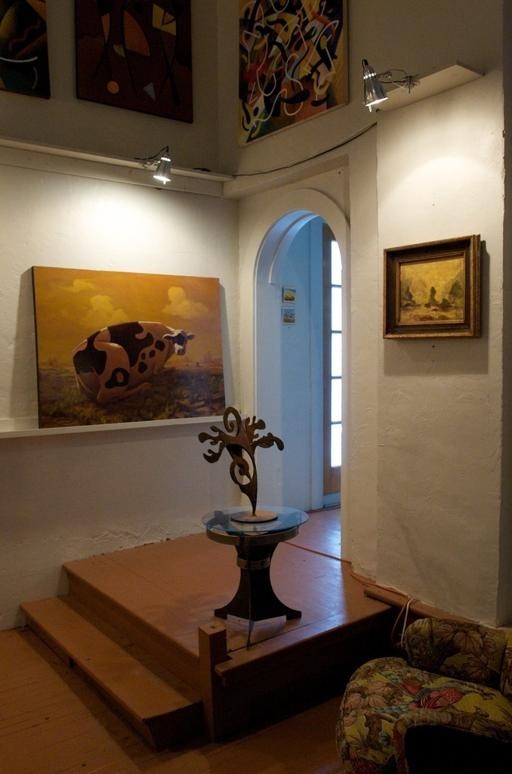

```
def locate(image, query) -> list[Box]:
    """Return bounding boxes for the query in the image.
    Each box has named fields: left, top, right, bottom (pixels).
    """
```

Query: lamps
left=360, top=58, right=420, bottom=107
left=142, top=145, right=171, bottom=183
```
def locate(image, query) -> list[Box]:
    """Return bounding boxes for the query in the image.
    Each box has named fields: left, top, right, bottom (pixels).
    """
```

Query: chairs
left=334, top=617, right=511, bottom=773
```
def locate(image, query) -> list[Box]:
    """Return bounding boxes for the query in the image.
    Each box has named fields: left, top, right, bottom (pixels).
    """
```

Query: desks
left=201, top=504, right=309, bottom=649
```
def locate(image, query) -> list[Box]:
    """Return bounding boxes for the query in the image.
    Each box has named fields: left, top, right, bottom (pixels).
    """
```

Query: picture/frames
left=281, top=306, right=297, bottom=324
left=282, top=286, right=296, bottom=303
left=382, top=234, right=483, bottom=338
left=237, top=0, right=349, bottom=150
left=74, top=0, right=194, bottom=125
